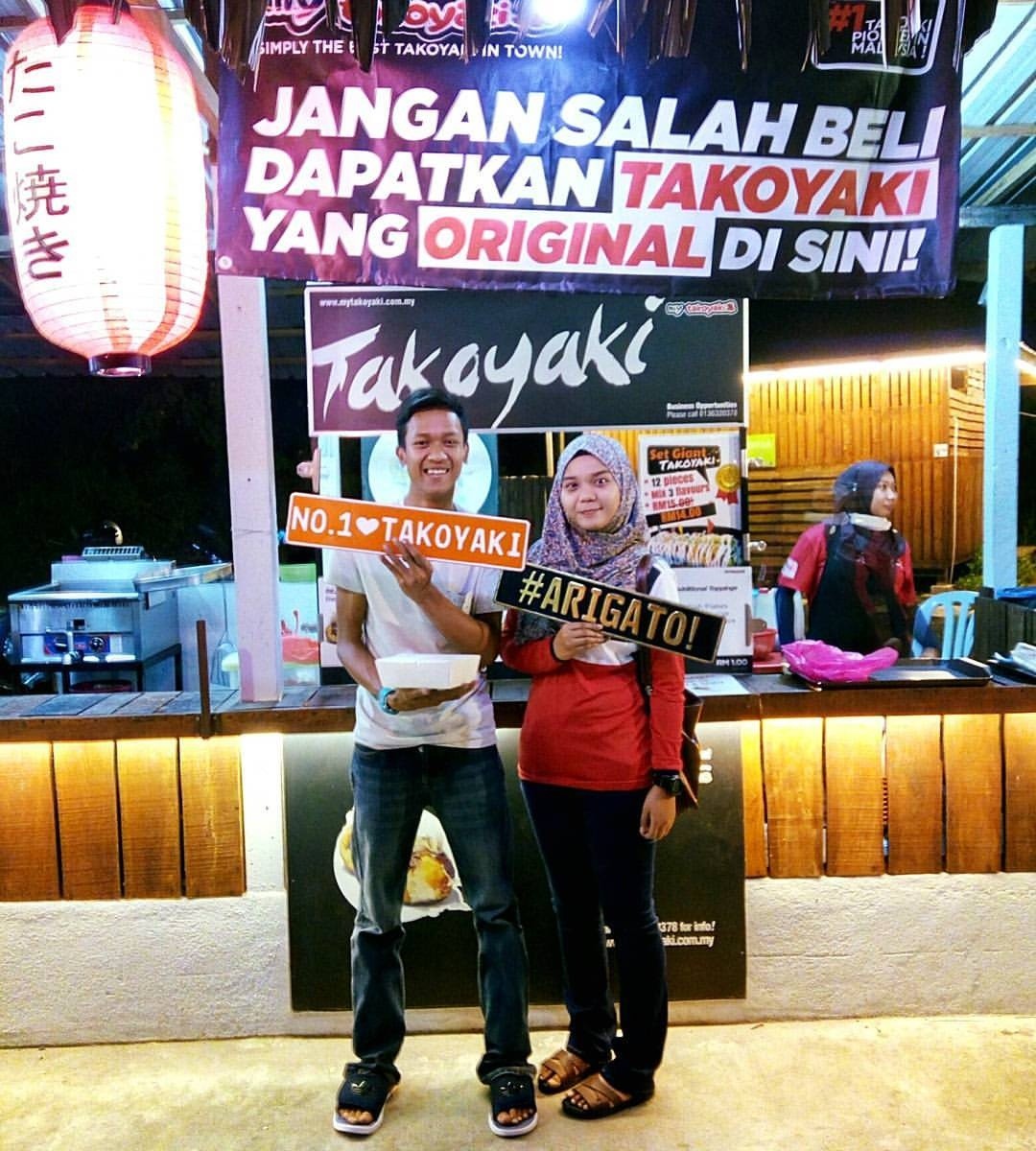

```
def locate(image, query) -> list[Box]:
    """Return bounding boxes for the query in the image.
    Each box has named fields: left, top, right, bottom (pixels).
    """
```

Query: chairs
left=911, top=589, right=981, bottom=659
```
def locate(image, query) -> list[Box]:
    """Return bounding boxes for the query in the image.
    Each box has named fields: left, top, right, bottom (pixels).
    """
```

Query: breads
left=339, top=810, right=454, bottom=903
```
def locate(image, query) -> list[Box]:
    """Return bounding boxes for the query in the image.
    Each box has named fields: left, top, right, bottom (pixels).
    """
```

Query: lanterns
left=3, top=2, right=214, bottom=379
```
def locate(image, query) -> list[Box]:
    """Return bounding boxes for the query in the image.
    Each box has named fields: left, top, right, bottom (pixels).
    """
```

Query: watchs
left=646, top=770, right=683, bottom=794
left=378, top=687, right=401, bottom=716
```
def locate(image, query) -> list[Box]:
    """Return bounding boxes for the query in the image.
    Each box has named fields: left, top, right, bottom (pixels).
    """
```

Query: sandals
left=562, top=1072, right=654, bottom=1121
left=489, top=1070, right=540, bottom=1136
left=332, top=1073, right=401, bottom=1134
left=535, top=1052, right=590, bottom=1095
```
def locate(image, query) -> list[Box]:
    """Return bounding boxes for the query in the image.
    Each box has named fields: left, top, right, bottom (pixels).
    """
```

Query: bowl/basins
left=753, top=628, right=777, bottom=661
left=67, top=679, right=132, bottom=692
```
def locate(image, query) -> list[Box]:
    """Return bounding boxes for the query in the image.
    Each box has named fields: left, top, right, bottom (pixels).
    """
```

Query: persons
left=773, top=459, right=944, bottom=660
left=498, top=433, right=685, bottom=1120
left=331, top=387, right=539, bottom=1139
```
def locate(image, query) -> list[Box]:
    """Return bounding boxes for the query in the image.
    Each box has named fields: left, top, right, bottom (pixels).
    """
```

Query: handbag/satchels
left=630, top=551, right=702, bottom=809
